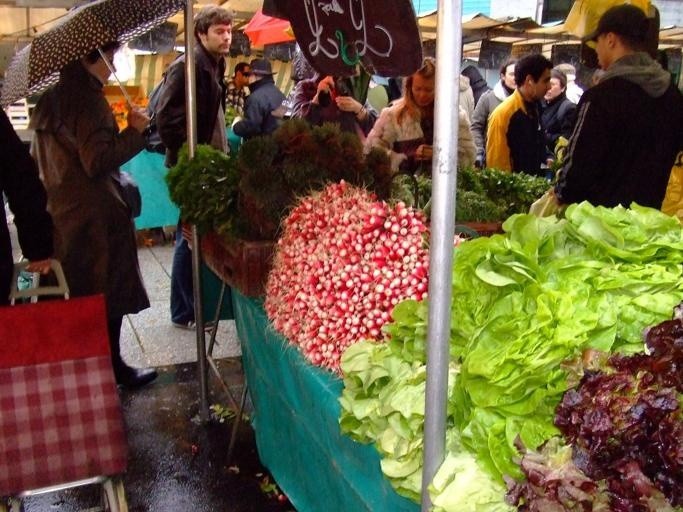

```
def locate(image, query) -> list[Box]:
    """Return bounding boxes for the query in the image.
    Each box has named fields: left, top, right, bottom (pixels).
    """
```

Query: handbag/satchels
left=118, top=171, right=142, bottom=218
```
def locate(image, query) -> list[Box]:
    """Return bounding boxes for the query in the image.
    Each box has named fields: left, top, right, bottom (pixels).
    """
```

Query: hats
left=555, top=63, right=576, bottom=80
left=243, top=58, right=277, bottom=75
left=582, top=4, right=649, bottom=42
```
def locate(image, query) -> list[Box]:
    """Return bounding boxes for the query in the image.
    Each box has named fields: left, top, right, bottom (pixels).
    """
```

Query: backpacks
left=143, top=54, right=201, bottom=152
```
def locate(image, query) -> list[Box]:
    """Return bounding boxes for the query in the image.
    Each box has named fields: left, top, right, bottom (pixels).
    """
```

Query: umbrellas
left=1, top=0, right=199, bottom=112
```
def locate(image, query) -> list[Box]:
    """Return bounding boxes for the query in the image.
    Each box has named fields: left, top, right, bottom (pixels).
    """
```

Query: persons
left=27, top=34, right=160, bottom=390
left=546, top=4, right=683, bottom=213
left=222, top=58, right=382, bottom=140
left=362, top=57, right=587, bottom=178
left=157, top=5, right=234, bottom=332
left=0, top=100, right=54, bottom=311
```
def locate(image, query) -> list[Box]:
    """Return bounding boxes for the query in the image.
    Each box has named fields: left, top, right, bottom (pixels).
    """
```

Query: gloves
left=474, top=152, right=485, bottom=168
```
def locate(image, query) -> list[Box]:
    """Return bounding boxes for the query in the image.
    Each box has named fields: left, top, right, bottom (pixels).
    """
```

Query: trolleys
left=0, top=260, right=130, bottom=512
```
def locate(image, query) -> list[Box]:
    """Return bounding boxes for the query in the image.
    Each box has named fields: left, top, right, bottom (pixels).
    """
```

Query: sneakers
left=173, top=320, right=214, bottom=331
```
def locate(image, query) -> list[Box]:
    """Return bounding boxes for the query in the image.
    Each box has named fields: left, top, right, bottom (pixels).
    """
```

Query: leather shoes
left=121, top=367, right=157, bottom=388
